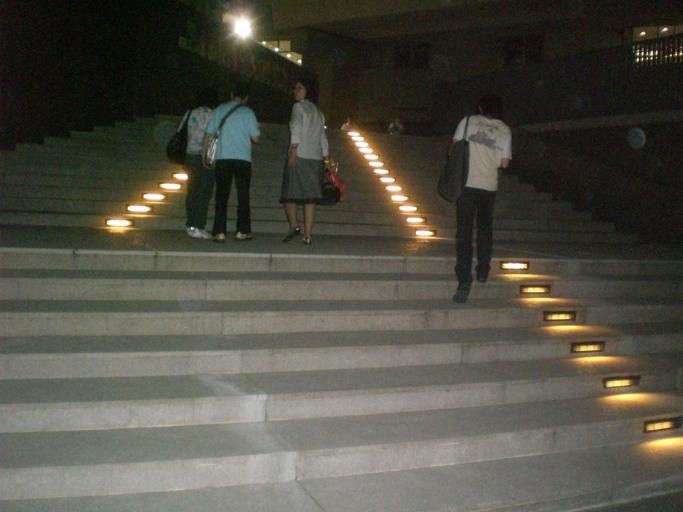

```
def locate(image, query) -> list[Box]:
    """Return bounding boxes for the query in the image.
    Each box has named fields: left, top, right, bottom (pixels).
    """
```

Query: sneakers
left=235, top=231, right=251, bottom=240
left=476, top=270, right=487, bottom=281
left=213, top=233, right=225, bottom=242
left=453, top=281, right=470, bottom=302
left=185, top=226, right=212, bottom=239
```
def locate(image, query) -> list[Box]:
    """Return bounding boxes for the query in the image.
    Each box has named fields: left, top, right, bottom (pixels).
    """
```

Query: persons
left=448, top=93, right=513, bottom=303
left=201, top=81, right=261, bottom=243
left=176, top=86, right=220, bottom=239
left=278, top=76, right=330, bottom=245
left=387, top=118, right=403, bottom=136
left=339, top=115, right=359, bottom=130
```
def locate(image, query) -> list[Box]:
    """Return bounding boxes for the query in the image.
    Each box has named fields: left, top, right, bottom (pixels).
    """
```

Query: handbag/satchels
left=205, top=137, right=218, bottom=166
left=437, top=139, right=468, bottom=202
left=315, top=170, right=345, bottom=205
left=166, top=122, right=187, bottom=163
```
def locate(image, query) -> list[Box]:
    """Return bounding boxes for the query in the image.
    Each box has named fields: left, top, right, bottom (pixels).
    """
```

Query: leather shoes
left=302, top=237, right=311, bottom=244
left=282, top=226, right=300, bottom=241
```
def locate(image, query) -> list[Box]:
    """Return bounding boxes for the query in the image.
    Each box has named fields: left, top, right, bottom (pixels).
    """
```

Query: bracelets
left=322, top=160, right=329, bottom=164
